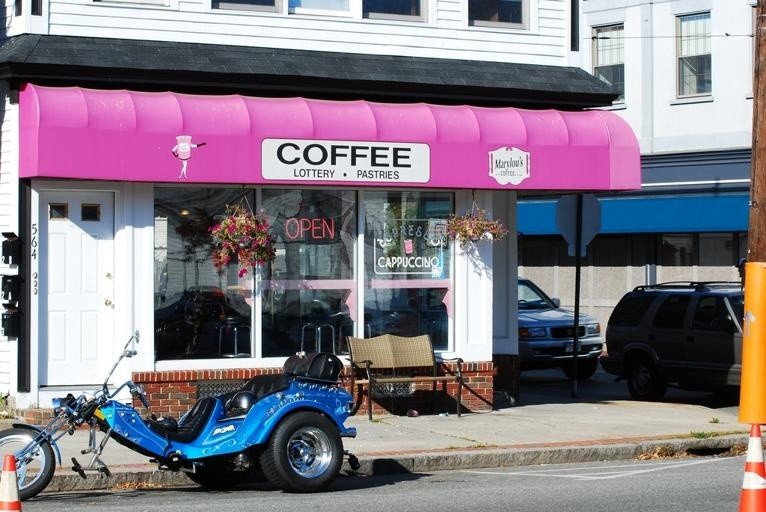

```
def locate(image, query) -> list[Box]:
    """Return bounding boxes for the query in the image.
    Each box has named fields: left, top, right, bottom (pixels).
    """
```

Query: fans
left=208, top=204, right=276, bottom=277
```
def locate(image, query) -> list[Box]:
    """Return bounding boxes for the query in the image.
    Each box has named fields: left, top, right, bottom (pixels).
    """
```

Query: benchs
left=346, top=334, right=464, bottom=421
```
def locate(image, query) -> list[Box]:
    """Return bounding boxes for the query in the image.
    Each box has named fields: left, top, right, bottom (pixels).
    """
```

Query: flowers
left=448, top=209, right=509, bottom=250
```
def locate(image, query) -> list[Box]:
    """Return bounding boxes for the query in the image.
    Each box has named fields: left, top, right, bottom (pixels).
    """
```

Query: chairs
left=224, top=351, right=341, bottom=418
left=149, top=396, right=221, bottom=444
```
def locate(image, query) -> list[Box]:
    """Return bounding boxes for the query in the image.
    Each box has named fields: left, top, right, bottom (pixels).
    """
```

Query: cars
left=518, top=277, right=603, bottom=380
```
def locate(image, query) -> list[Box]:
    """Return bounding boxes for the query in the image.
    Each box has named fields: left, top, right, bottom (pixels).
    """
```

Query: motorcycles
left=0, top=330, right=360, bottom=502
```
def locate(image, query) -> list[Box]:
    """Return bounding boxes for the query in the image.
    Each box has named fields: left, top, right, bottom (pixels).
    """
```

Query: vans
left=600, top=281, right=745, bottom=401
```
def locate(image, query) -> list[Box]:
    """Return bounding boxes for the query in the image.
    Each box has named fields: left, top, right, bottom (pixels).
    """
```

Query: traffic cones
left=0, top=454, right=21, bottom=512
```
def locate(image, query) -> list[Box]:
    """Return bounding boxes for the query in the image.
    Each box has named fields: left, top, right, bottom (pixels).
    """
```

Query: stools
left=220, top=317, right=250, bottom=357
left=300, top=321, right=335, bottom=356
left=339, top=320, right=371, bottom=353
